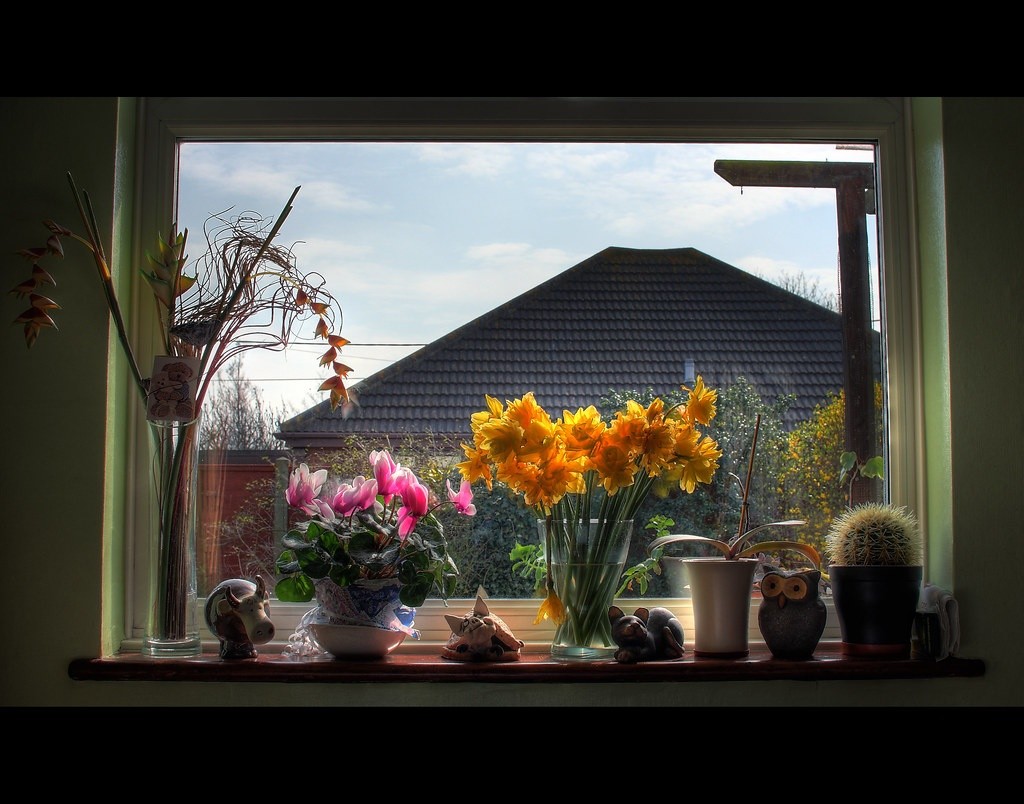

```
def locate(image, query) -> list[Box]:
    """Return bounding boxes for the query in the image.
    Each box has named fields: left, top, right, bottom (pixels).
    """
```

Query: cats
left=608, top=605, right=685, bottom=665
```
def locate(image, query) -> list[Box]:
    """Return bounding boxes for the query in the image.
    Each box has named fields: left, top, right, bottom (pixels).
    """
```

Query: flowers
left=273, top=455, right=477, bottom=606
left=458, top=376, right=722, bottom=648
left=4, top=167, right=360, bottom=639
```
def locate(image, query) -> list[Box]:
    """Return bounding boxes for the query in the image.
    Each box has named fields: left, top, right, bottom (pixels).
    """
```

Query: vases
left=535, top=517, right=634, bottom=659
left=139, top=419, right=204, bottom=657
left=311, top=571, right=405, bottom=631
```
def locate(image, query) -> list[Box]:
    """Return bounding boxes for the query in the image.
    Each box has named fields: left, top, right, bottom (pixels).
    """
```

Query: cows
left=205, top=575, right=275, bottom=660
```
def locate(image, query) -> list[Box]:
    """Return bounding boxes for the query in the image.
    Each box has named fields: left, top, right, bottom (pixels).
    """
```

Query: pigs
left=443, top=594, right=525, bottom=653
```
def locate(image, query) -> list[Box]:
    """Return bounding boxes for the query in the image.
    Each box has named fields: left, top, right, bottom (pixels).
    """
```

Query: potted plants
left=646, top=473, right=821, bottom=657
left=823, top=503, right=924, bottom=661
